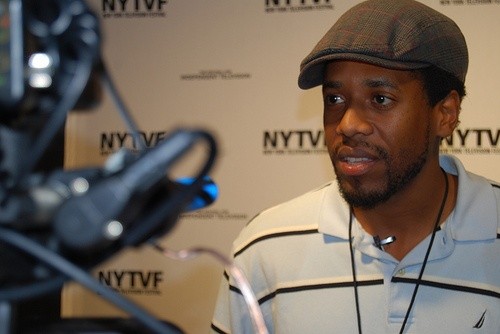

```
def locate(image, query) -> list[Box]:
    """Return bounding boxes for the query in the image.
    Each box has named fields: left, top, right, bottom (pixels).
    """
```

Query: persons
left=211, top=0, right=500, bottom=334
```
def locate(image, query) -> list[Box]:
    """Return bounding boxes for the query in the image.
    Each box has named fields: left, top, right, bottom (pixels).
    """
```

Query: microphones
left=54, top=130, right=192, bottom=246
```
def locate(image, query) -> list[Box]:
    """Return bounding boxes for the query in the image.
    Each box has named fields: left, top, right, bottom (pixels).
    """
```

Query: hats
left=297, top=0, right=469, bottom=91
